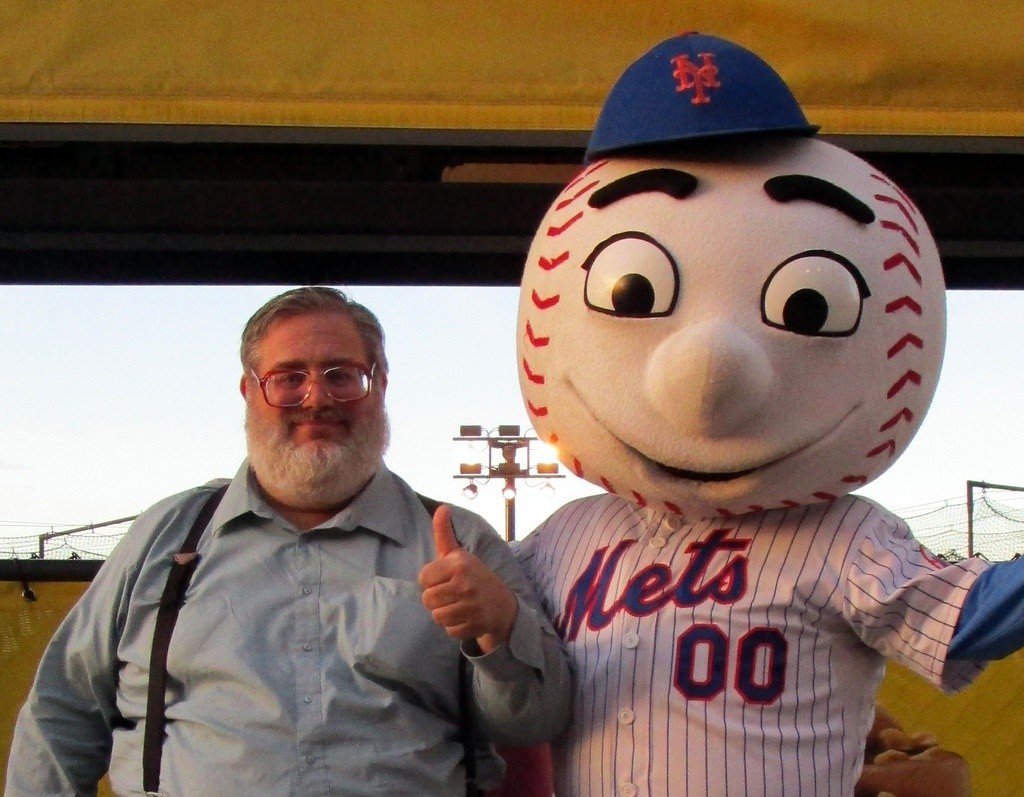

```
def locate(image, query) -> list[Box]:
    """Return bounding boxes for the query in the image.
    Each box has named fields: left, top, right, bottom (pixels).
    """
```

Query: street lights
left=449, top=426, right=567, bottom=544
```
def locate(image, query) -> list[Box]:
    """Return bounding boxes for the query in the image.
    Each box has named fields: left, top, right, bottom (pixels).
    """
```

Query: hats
left=582, top=32, right=821, bottom=157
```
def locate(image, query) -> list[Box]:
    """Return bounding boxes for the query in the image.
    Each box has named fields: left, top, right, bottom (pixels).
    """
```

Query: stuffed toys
left=505, top=32, right=1023, bottom=797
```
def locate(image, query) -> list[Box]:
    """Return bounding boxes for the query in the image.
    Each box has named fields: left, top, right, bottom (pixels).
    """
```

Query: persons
left=3, top=288, right=571, bottom=797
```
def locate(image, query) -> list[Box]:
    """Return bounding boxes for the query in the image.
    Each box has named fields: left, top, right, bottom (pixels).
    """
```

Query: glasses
left=250, top=362, right=377, bottom=407
